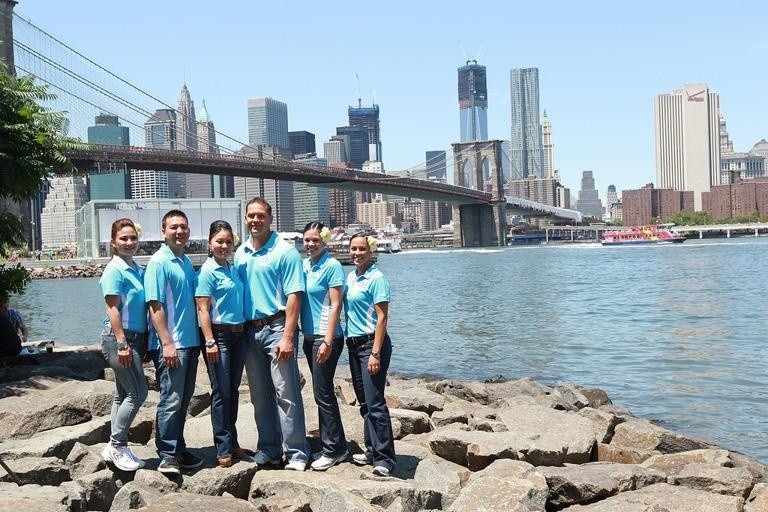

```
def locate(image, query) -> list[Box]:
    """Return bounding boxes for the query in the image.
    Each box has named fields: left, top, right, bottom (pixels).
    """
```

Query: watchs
left=204, top=340, right=216, bottom=347
left=323, top=339, right=333, bottom=347
left=371, top=350, right=381, bottom=358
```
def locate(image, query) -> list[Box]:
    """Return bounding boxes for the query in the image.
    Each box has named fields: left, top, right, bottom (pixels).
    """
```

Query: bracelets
left=115, top=342, right=129, bottom=349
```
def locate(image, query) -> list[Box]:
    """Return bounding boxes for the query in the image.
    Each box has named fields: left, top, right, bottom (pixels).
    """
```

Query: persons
left=192, top=220, right=247, bottom=469
left=35, top=246, right=76, bottom=260
left=99, top=218, right=150, bottom=472
left=302, top=221, right=350, bottom=472
left=233, top=196, right=311, bottom=471
left=341, top=233, right=397, bottom=477
left=144, top=208, right=204, bottom=477
left=0, top=297, right=28, bottom=358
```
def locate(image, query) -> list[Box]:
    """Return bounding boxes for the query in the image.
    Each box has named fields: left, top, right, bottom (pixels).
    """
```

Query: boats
left=599, top=223, right=688, bottom=246
left=322, top=223, right=401, bottom=267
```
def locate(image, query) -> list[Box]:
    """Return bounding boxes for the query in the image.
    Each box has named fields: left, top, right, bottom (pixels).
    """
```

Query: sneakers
left=101, top=442, right=397, bottom=477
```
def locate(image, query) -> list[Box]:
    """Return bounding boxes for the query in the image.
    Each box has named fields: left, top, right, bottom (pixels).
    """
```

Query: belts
left=213, top=324, right=244, bottom=332
left=347, top=335, right=374, bottom=346
left=246, top=312, right=284, bottom=328
